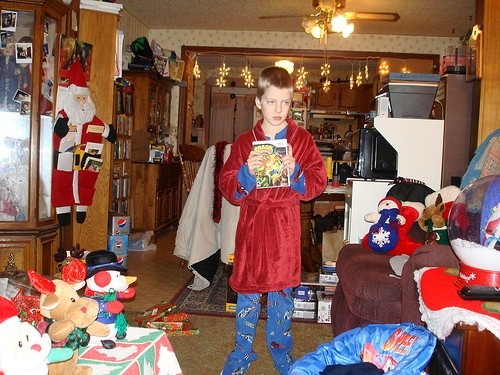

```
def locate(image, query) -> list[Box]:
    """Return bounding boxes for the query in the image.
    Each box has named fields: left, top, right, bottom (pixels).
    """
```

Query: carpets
left=169, top=262, right=324, bottom=324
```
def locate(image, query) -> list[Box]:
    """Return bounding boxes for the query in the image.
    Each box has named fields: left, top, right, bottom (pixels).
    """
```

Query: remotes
left=462, top=285, right=500, bottom=295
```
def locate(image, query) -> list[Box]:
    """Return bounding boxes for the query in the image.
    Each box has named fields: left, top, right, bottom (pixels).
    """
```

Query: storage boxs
left=108, top=214, right=130, bottom=236
left=108, top=235, right=128, bottom=254
left=292, top=265, right=339, bottom=323
left=226, top=295, right=238, bottom=312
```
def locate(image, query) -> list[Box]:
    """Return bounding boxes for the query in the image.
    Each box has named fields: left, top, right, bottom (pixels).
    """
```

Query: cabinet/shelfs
left=315, top=84, right=360, bottom=111
left=0, top=0, right=69, bottom=278
left=108, top=70, right=182, bottom=243
left=440, top=323, right=500, bottom=375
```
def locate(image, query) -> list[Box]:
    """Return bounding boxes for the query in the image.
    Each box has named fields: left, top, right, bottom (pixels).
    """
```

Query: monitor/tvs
left=360, top=128, right=398, bottom=180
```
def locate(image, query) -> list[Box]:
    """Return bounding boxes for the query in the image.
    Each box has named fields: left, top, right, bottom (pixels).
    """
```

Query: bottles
left=308, top=121, right=342, bottom=140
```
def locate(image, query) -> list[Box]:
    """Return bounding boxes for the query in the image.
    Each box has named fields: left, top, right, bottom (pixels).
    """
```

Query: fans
left=259, top=0, right=400, bottom=33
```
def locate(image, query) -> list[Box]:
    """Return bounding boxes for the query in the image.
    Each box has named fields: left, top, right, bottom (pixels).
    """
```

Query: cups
left=325, top=156, right=340, bottom=187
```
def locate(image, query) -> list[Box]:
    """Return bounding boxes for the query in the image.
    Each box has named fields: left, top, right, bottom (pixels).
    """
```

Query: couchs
left=330, top=239, right=461, bottom=337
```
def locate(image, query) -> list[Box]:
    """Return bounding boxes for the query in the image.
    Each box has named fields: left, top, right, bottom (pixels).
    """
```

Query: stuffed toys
left=418, top=193, right=448, bottom=245
left=364, top=197, right=406, bottom=253
left=0, top=250, right=137, bottom=375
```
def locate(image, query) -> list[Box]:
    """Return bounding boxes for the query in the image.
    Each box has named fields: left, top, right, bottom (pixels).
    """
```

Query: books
left=252, top=139, right=291, bottom=189
left=113, top=92, right=132, bottom=213
left=57, top=124, right=104, bottom=172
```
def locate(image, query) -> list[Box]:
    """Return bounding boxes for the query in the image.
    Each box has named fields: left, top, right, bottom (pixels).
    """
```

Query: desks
left=69, top=326, right=184, bottom=375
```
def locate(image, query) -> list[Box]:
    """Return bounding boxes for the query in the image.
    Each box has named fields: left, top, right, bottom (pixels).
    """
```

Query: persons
left=219, top=66, right=328, bottom=375
left=3, top=14, right=12, bottom=26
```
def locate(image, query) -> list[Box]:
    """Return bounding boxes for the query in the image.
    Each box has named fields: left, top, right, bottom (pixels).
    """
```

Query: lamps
left=302, top=15, right=355, bottom=46
left=194, top=55, right=412, bottom=94
